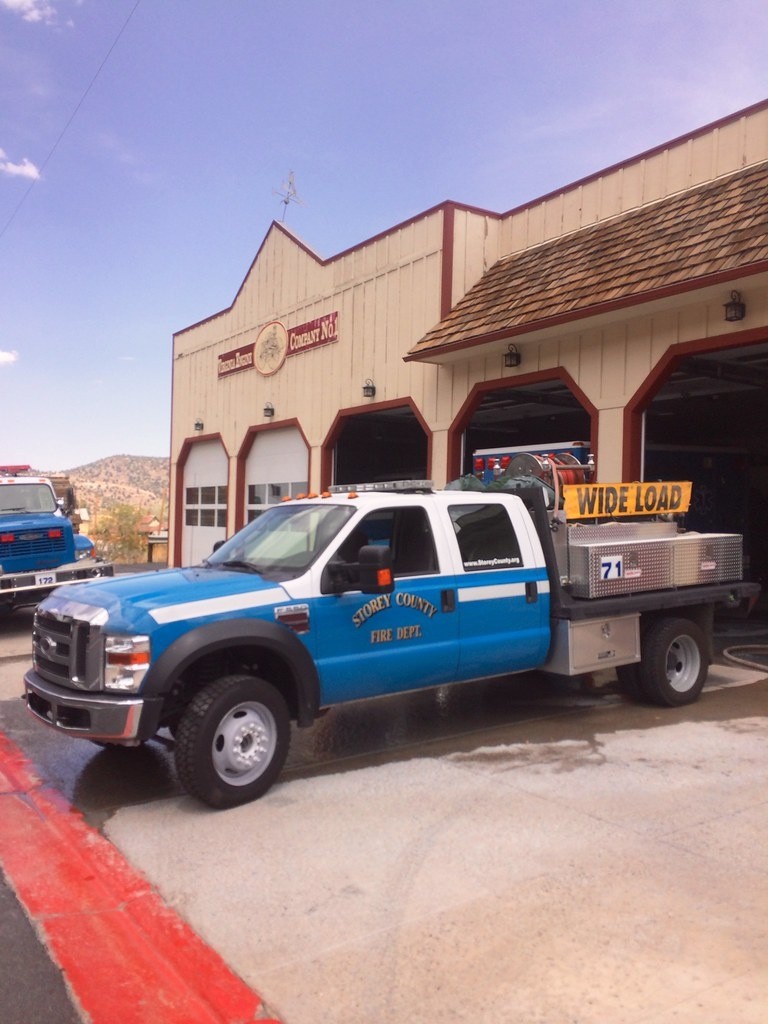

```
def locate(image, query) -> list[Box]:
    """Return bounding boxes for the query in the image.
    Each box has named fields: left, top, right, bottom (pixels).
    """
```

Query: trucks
left=0, top=464, right=114, bottom=625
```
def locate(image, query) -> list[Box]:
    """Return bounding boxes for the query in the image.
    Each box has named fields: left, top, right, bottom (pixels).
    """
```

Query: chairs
left=321, top=529, right=368, bottom=564
left=389, top=509, right=437, bottom=574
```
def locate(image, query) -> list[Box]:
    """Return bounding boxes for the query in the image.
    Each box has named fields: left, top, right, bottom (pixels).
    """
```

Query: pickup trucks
left=23, top=481, right=765, bottom=811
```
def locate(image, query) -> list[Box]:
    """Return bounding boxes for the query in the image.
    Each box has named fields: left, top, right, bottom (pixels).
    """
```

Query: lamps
left=362, top=378, right=375, bottom=397
left=722, top=290, right=746, bottom=322
left=263, top=402, right=274, bottom=417
left=194, top=418, right=203, bottom=431
left=502, top=344, right=521, bottom=367
